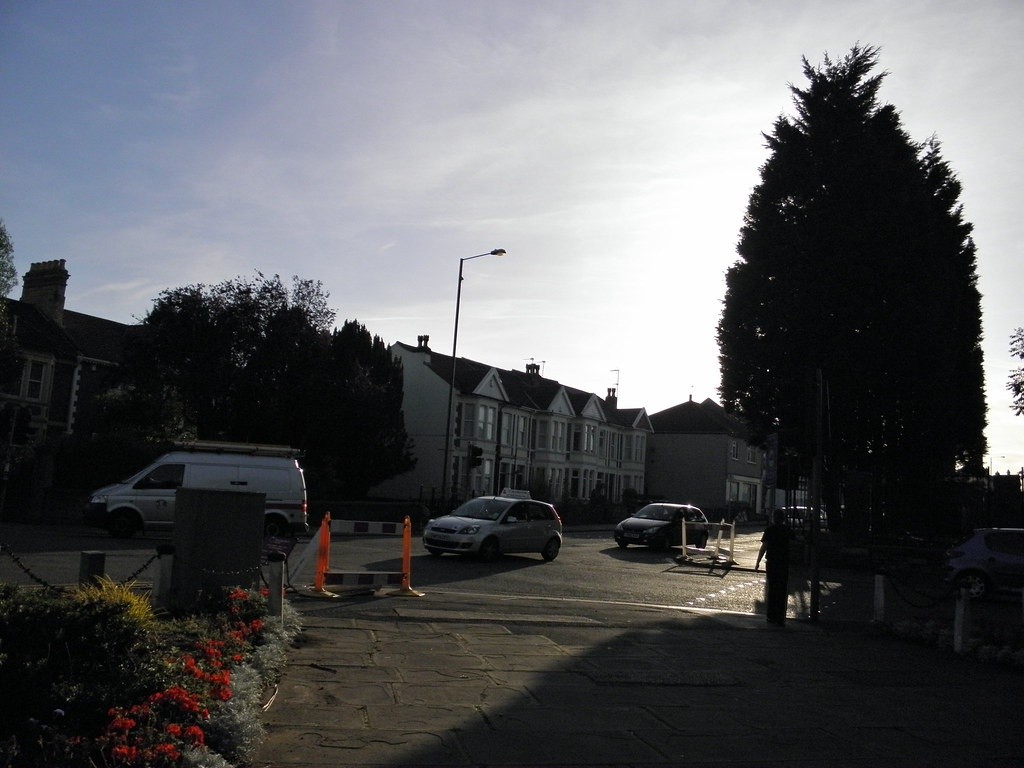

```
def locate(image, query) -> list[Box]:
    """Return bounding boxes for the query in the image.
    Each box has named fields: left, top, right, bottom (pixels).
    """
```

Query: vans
left=88, top=437, right=308, bottom=541
left=782, top=506, right=827, bottom=529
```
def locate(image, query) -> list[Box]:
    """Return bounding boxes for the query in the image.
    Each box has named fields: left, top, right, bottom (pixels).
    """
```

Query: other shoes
left=766, top=617, right=784, bottom=626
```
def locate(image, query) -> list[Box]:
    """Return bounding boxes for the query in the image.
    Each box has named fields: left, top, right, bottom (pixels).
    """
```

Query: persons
left=756, top=509, right=795, bottom=627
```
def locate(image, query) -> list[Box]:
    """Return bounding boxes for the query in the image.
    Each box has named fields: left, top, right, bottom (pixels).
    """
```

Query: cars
left=614, top=502, right=709, bottom=553
left=422, top=494, right=564, bottom=561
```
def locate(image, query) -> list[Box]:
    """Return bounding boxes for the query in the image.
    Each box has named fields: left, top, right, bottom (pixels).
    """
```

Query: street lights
left=440, top=249, right=506, bottom=505
